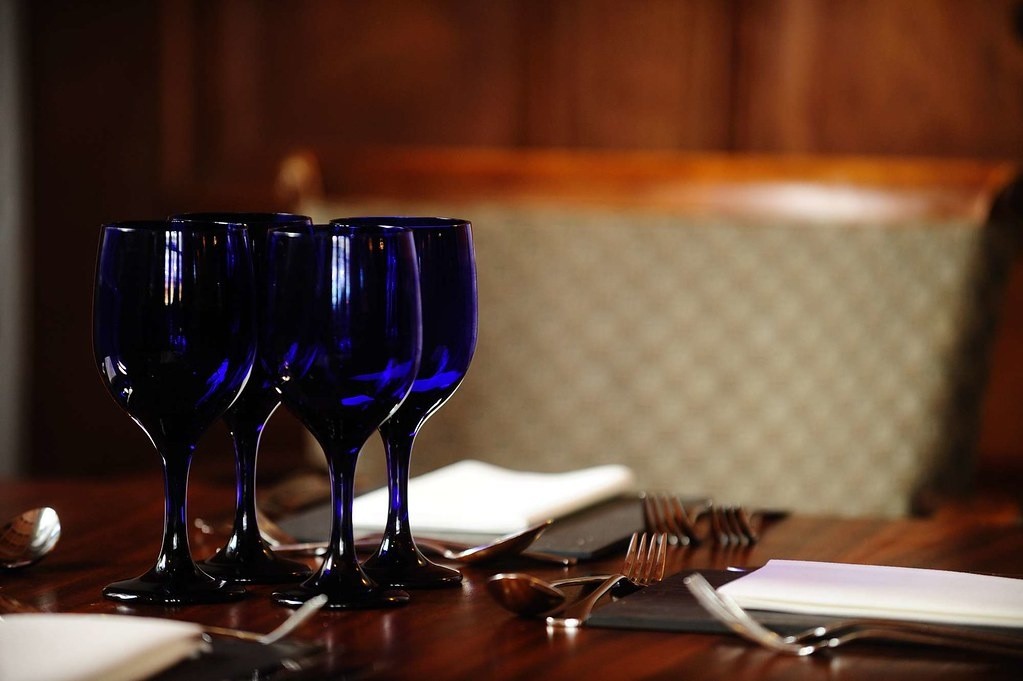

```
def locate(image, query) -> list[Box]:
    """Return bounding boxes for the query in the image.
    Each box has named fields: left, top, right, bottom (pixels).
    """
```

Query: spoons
left=486, top=568, right=618, bottom=617
left=0, top=504, right=63, bottom=572
left=268, top=517, right=554, bottom=561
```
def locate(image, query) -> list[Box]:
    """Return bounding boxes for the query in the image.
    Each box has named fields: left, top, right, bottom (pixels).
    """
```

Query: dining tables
left=0, top=473, right=1023, bottom=681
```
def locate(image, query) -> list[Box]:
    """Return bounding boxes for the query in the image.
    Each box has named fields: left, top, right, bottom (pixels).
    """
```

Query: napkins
left=350, top=459, right=634, bottom=549
left=0, top=608, right=216, bottom=681
left=717, top=556, right=1023, bottom=636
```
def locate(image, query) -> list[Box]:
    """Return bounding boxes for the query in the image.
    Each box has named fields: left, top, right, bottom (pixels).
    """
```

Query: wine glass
left=271, top=220, right=422, bottom=610
left=169, top=211, right=317, bottom=585
left=341, top=216, right=479, bottom=591
left=94, top=222, right=250, bottom=601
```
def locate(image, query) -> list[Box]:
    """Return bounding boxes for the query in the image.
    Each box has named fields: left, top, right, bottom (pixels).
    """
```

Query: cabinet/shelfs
left=24, top=0, right=1023, bottom=501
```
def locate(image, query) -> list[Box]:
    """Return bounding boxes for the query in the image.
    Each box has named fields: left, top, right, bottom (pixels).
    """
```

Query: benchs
left=239, top=151, right=1023, bottom=503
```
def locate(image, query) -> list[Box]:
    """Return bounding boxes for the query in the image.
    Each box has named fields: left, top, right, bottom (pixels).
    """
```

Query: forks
left=683, top=570, right=1023, bottom=668
left=640, top=489, right=787, bottom=548
left=545, top=532, right=668, bottom=628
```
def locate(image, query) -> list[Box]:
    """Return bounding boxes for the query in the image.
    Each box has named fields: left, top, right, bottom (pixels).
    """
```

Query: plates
left=715, top=558, right=1020, bottom=652
